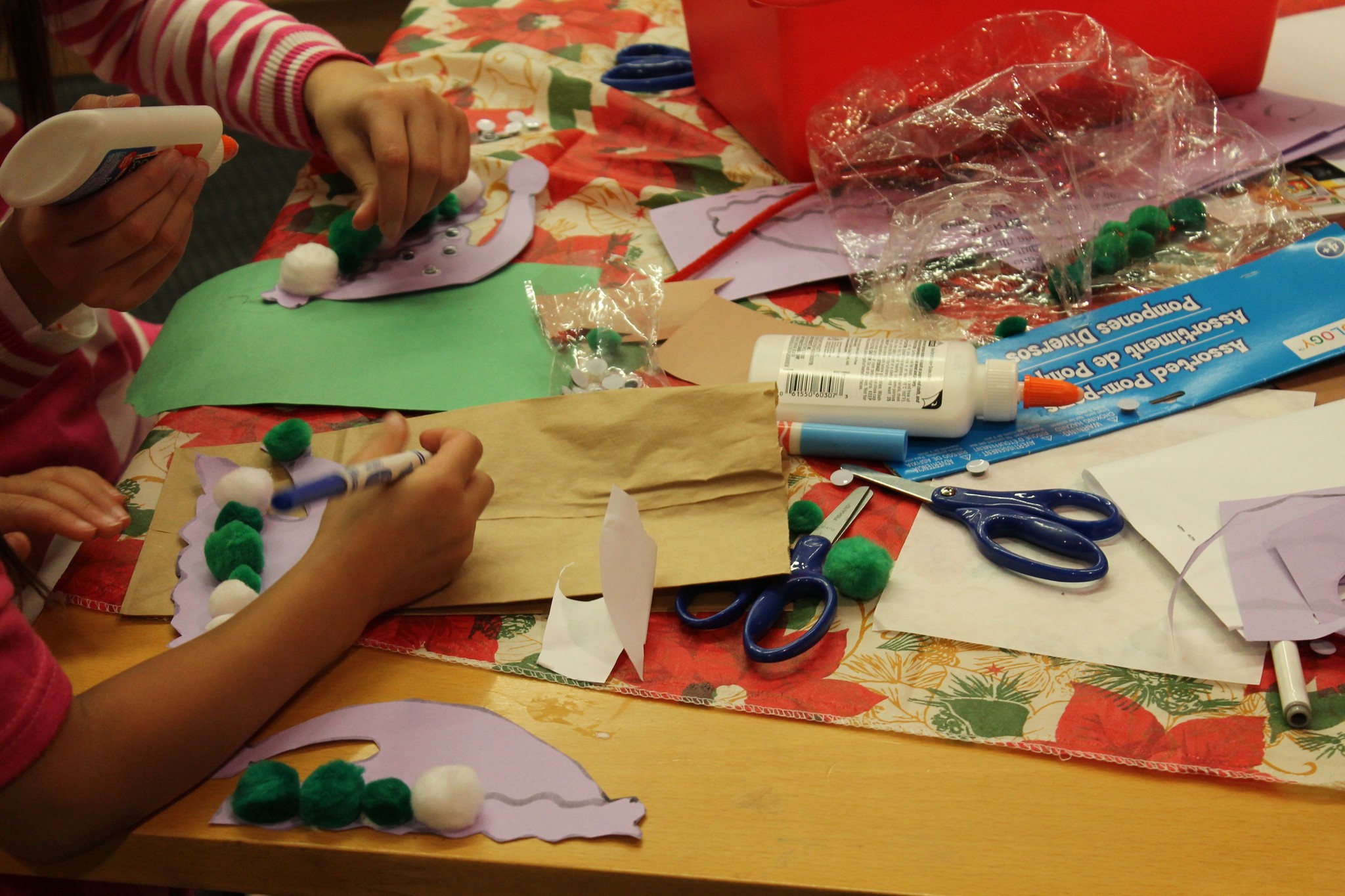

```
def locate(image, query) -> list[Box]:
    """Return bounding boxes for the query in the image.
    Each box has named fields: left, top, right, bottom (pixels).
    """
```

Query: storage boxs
left=680, top=0, right=1280, bottom=193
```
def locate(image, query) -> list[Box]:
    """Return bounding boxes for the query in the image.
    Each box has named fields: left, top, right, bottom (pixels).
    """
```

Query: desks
left=1, top=0, right=1343, bottom=896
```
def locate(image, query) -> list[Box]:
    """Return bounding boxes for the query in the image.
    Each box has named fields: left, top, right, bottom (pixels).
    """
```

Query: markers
left=776, top=419, right=910, bottom=465
left=270, top=447, right=434, bottom=512
left=1270, top=640, right=1312, bottom=731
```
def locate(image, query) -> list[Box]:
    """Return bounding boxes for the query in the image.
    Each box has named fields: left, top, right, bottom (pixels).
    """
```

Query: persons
left=1, top=398, right=495, bottom=896
left=0, top=0, right=471, bottom=476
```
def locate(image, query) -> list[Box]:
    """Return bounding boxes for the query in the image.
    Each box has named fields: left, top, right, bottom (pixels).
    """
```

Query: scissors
left=674, top=485, right=874, bottom=663
left=840, top=463, right=1125, bottom=584
left=600, top=41, right=695, bottom=92
